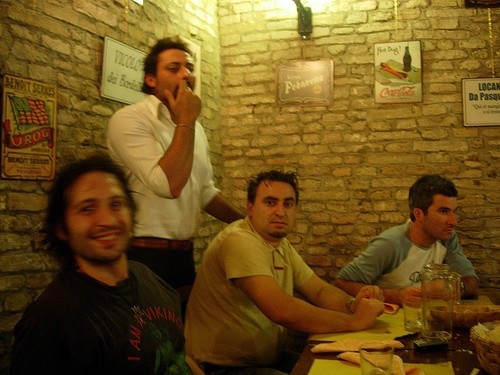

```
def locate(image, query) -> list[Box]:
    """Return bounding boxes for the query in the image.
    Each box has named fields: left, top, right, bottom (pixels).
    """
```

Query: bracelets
left=347, top=299, right=355, bottom=313
left=178, top=123, right=194, bottom=131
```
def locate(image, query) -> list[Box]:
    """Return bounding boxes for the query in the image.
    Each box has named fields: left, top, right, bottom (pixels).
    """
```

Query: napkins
left=311, top=335, right=405, bottom=353
left=383, top=302, right=399, bottom=314
left=336, top=351, right=425, bottom=375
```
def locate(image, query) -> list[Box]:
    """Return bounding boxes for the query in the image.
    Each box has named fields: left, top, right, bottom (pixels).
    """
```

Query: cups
left=402, top=296, right=423, bottom=332
left=358, top=341, right=395, bottom=375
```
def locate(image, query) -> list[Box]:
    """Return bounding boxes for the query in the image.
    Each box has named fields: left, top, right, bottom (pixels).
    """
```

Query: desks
left=289, top=296, right=500, bottom=375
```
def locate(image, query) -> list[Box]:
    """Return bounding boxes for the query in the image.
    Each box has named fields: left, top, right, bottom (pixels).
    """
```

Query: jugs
left=420, top=264, right=464, bottom=343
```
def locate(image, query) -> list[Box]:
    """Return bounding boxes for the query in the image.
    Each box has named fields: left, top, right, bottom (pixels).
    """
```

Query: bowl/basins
left=471, top=321, right=500, bottom=375
left=431, top=303, right=500, bottom=328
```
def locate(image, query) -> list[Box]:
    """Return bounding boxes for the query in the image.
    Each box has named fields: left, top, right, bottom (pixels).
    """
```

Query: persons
left=8, top=153, right=205, bottom=375
left=107, top=37, right=246, bottom=296
left=334, top=174, right=479, bottom=307
left=184, top=170, right=385, bottom=375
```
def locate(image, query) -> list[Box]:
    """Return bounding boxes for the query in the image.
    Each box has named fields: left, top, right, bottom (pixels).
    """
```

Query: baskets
left=470, top=319, right=500, bottom=375
left=430, top=305, right=500, bottom=327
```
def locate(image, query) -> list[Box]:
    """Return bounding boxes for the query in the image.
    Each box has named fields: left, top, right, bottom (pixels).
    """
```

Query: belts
left=128, top=237, right=193, bottom=250
